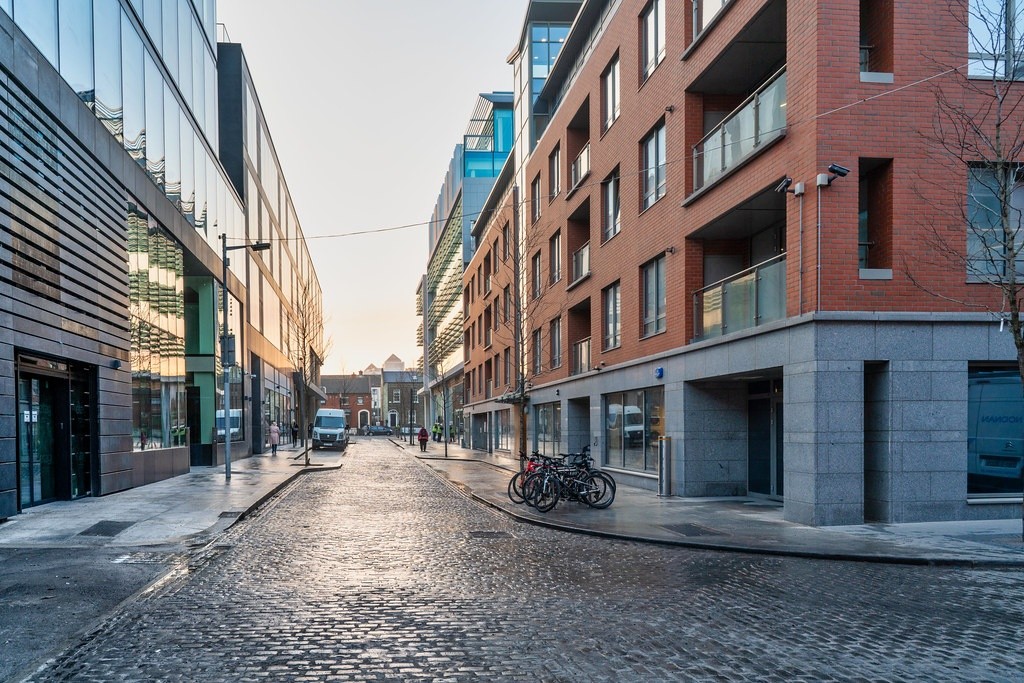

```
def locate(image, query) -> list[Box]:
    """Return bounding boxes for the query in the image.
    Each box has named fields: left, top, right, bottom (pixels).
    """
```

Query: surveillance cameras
left=828, top=164, right=851, bottom=177
left=774, top=178, right=792, bottom=193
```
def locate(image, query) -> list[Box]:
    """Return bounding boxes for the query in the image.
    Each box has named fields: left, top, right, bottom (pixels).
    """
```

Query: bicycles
left=507, top=445, right=616, bottom=513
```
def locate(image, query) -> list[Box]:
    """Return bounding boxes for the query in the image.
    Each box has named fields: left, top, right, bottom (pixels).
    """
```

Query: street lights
left=224, top=232, right=272, bottom=478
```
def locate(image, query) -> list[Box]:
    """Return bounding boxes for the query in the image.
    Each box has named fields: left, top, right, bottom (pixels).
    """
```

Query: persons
left=139, top=429, right=146, bottom=449
left=307, top=421, right=313, bottom=438
left=172, top=426, right=186, bottom=447
left=268, top=422, right=280, bottom=456
left=396, top=422, right=444, bottom=452
left=450, top=424, right=456, bottom=442
left=291, top=421, right=299, bottom=446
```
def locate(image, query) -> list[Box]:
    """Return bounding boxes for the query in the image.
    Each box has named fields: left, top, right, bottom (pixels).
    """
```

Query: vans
left=607, top=403, right=660, bottom=447
left=216, top=408, right=244, bottom=442
left=310, top=408, right=351, bottom=451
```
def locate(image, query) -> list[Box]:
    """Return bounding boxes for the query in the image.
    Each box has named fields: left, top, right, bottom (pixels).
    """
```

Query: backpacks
left=420, top=429, right=426, bottom=436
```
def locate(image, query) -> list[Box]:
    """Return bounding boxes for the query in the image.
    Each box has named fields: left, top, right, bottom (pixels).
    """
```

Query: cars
left=367, top=425, right=392, bottom=436
